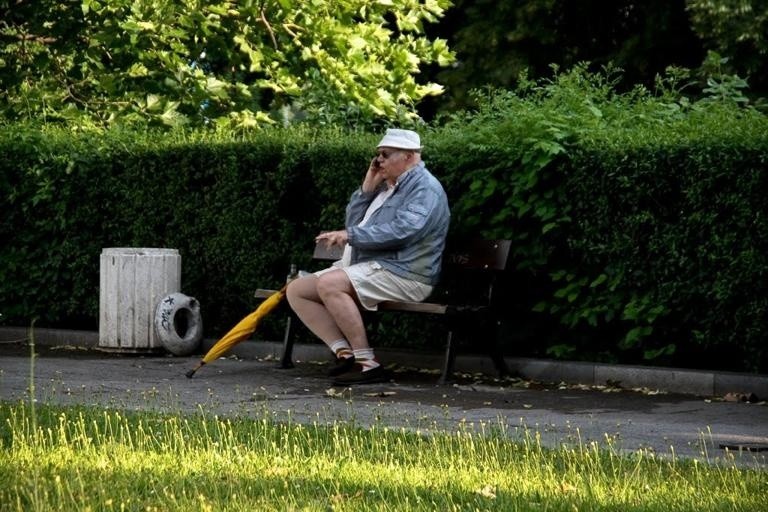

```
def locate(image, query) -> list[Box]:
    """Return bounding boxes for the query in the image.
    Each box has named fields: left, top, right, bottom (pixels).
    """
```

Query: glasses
left=375, top=150, right=395, bottom=159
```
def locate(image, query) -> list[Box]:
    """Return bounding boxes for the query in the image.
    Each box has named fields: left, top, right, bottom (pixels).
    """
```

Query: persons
left=284, top=127, right=450, bottom=385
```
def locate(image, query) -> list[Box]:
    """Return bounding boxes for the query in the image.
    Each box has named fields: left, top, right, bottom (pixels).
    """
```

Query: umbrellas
left=183, top=285, right=284, bottom=381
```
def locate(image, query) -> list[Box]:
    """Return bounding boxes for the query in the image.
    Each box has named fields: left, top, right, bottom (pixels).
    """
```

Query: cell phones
left=374, top=158, right=379, bottom=169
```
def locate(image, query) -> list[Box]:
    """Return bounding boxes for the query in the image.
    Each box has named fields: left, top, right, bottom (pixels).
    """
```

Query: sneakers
left=327, top=353, right=356, bottom=377
left=334, top=364, right=387, bottom=385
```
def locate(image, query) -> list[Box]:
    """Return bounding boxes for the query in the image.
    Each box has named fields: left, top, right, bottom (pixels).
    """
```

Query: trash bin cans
left=98, top=247, right=181, bottom=353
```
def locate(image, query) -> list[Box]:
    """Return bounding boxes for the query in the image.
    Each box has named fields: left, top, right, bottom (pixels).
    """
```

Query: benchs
left=254, top=231, right=515, bottom=386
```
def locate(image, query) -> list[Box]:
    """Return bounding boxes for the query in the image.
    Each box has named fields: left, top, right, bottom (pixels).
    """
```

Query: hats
left=375, top=127, right=421, bottom=150
